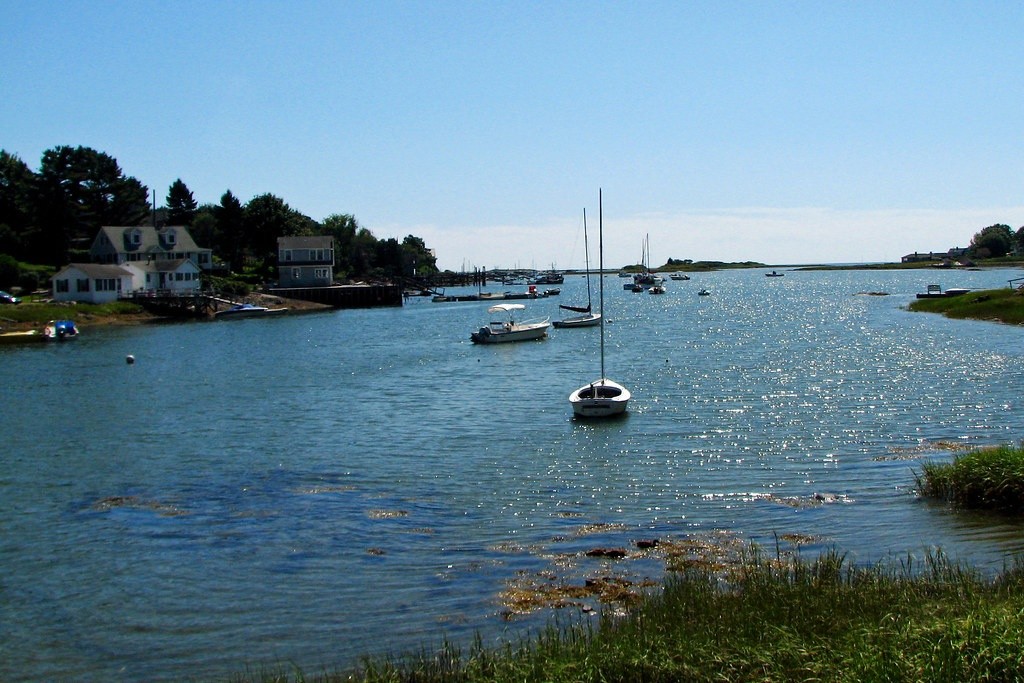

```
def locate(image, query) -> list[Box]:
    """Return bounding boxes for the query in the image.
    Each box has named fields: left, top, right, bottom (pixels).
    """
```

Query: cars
left=0, top=291, right=22, bottom=305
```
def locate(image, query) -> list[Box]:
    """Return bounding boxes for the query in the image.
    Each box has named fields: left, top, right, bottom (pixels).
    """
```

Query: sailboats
left=552, top=208, right=601, bottom=329
left=568, top=188, right=631, bottom=416
left=634, top=233, right=667, bottom=286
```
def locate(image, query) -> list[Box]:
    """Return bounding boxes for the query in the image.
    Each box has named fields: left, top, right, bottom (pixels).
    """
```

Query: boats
left=916, top=285, right=970, bottom=299
left=648, top=286, right=666, bottom=294
left=618, top=270, right=631, bottom=277
left=478, top=271, right=564, bottom=302
left=765, top=273, right=785, bottom=277
left=698, top=290, right=711, bottom=296
left=670, top=271, right=690, bottom=280
left=469, top=304, right=551, bottom=345
left=623, top=283, right=644, bottom=293
left=215, top=304, right=268, bottom=319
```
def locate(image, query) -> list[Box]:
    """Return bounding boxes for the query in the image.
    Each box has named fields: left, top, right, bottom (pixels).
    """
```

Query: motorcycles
left=44, top=320, right=79, bottom=342
